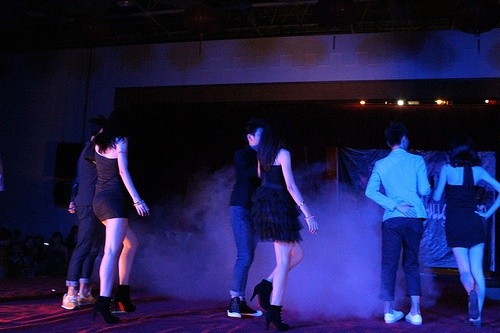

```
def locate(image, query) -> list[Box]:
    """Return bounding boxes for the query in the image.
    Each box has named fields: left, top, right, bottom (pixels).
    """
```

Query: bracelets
left=133, top=200, right=145, bottom=206
left=303, top=215, right=316, bottom=219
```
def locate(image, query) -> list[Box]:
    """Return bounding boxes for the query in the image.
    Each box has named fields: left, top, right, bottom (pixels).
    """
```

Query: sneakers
left=61, top=294, right=81, bottom=310
left=78, top=291, right=97, bottom=306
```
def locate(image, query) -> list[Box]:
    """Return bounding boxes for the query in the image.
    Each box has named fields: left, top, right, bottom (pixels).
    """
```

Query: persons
left=364, top=126, right=500, bottom=328
left=61, top=101, right=150, bottom=326
left=226, top=118, right=319, bottom=332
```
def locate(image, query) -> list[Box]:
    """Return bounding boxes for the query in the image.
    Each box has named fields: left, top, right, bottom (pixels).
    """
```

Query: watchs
left=298, top=201, right=305, bottom=208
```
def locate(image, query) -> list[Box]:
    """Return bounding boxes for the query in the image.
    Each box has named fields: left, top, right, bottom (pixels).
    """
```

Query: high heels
left=93, top=296, right=120, bottom=324
left=250, top=279, right=274, bottom=310
left=264, top=304, right=290, bottom=331
left=114, top=285, right=136, bottom=312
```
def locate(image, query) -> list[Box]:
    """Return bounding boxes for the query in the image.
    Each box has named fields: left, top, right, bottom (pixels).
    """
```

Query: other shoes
left=468, top=290, right=480, bottom=318
left=384, top=309, right=404, bottom=323
left=463, top=317, right=482, bottom=330
left=227, top=301, right=263, bottom=318
left=405, top=312, right=422, bottom=325
